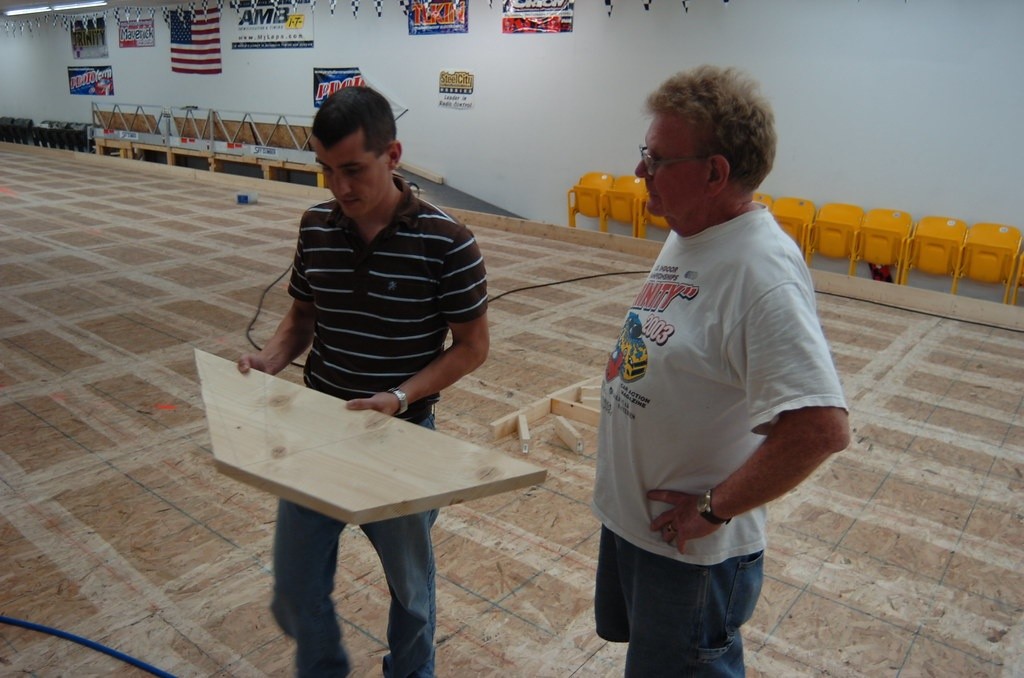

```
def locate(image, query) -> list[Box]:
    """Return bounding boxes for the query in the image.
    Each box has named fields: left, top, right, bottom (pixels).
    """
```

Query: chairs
left=566, top=172, right=1024, bottom=305
left=0, top=117, right=102, bottom=154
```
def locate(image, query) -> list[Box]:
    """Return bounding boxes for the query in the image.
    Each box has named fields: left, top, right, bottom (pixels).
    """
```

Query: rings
left=667, top=523, right=678, bottom=533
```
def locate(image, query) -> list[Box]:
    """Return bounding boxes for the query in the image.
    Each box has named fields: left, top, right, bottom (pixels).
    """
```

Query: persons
left=238, top=86, right=490, bottom=678
left=592, top=62, right=851, bottom=678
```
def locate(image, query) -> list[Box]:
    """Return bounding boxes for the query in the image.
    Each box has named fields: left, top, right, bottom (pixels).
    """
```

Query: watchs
left=697, top=489, right=732, bottom=525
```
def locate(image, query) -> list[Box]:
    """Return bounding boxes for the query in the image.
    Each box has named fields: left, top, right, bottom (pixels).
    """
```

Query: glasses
left=639, top=144, right=708, bottom=169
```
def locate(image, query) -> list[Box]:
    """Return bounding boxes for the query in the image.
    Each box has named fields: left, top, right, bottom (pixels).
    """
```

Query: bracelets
left=387, top=387, right=409, bottom=416
